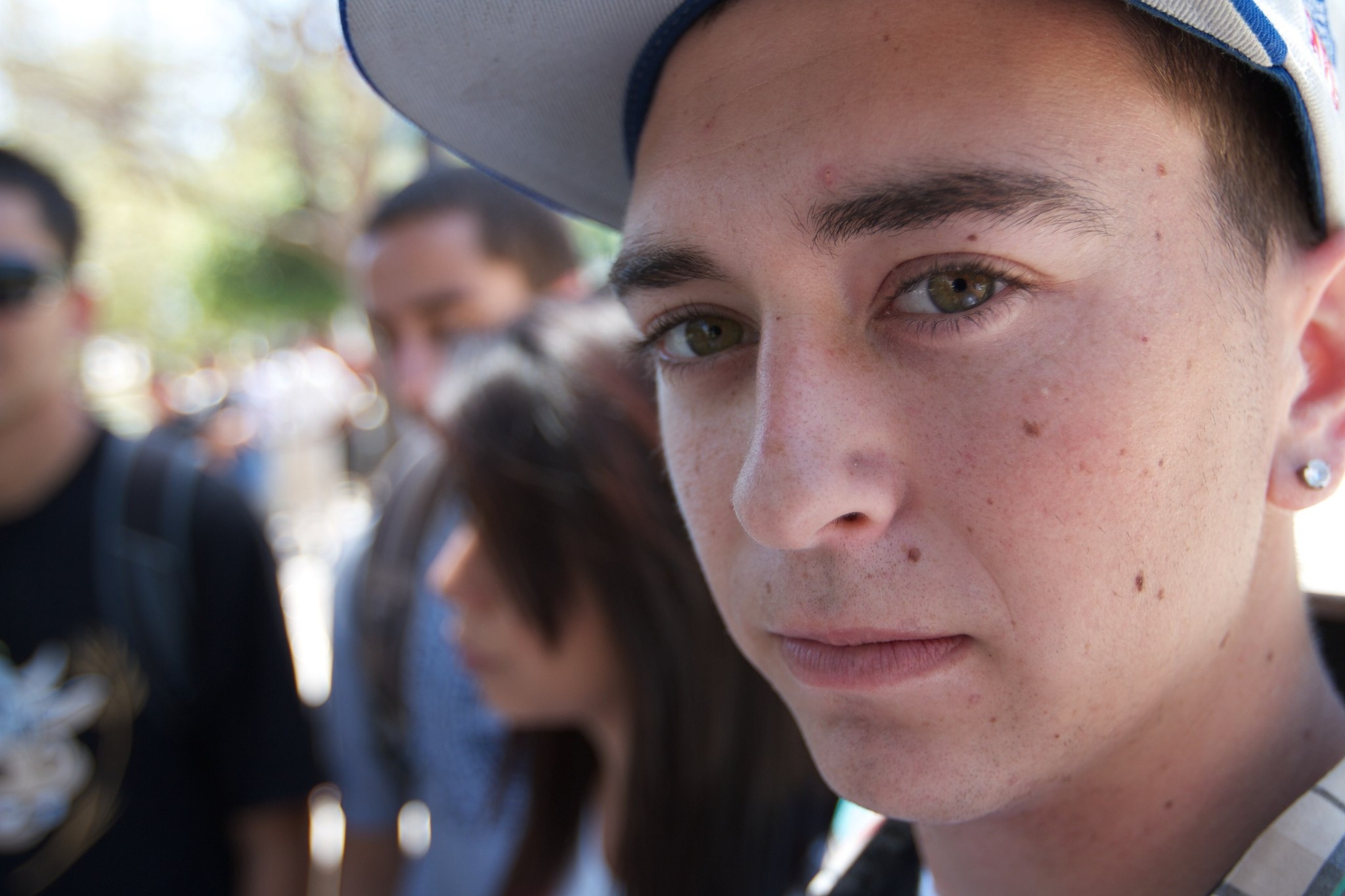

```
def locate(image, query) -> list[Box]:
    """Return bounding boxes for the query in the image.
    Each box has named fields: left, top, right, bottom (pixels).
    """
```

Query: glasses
left=1, top=257, right=60, bottom=307
left=509, top=295, right=673, bottom=454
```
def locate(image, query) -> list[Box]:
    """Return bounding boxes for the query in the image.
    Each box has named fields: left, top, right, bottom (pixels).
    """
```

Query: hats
left=334, top=0, right=1345, bottom=235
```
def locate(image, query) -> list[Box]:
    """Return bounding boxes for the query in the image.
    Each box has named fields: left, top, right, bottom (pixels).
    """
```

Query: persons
left=342, top=1, right=1344, bottom=894
left=422, top=298, right=836, bottom=896
left=0, top=152, right=313, bottom=896
left=320, top=168, right=585, bottom=896
